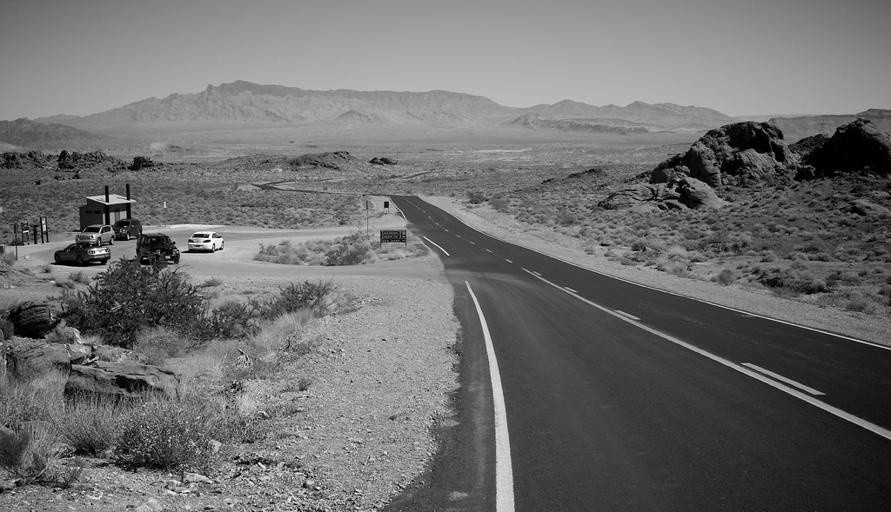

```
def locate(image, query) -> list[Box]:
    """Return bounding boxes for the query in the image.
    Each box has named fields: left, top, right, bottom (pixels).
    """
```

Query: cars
left=136, top=233, right=180, bottom=264
left=188, top=231, right=224, bottom=253
left=54, top=243, right=111, bottom=267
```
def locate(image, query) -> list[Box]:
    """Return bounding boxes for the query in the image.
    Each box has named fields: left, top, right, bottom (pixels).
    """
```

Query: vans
left=76, top=219, right=143, bottom=247
